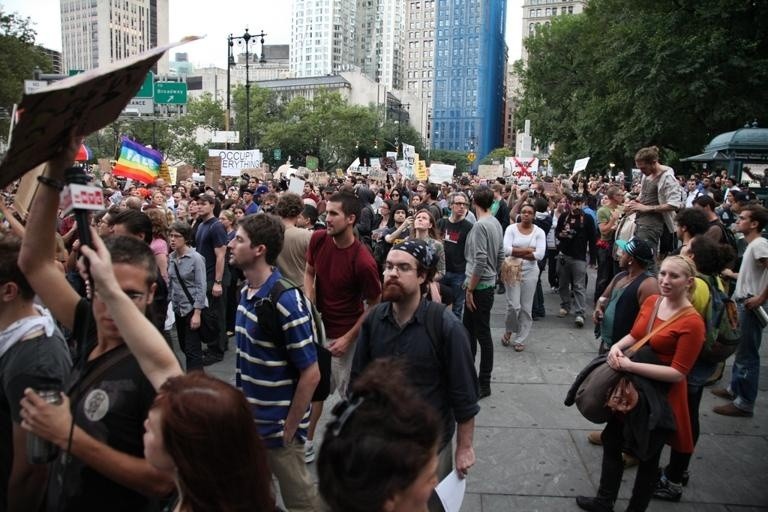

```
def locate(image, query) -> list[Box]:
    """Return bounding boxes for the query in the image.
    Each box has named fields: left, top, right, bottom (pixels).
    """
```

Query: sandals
left=514, top=342, right=525, bottom=351
left=502, top=332, right=511, bottom=345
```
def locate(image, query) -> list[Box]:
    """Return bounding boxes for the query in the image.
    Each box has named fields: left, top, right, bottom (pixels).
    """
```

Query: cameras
left=563, top=230, right=575, bottom=236
left=527, top=191, right=535, bottom=196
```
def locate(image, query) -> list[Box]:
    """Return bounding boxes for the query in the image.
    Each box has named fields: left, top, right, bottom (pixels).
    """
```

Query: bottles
left=25, top=384, right=63, bottom=465
left=745, top=293, right=768, bottom=328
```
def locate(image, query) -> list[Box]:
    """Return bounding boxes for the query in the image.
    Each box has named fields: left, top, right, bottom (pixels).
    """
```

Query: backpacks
left=695, top=273, right=743, bottom=362
left=265, top=278, right=332, bottom=401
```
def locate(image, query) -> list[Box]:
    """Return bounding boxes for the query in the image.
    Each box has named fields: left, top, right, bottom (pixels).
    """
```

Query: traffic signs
left=153, top=82, right=186, bottom=105
left=274, top=149, right=283, bottom=160
left=69, top=68, right=155, bottom=116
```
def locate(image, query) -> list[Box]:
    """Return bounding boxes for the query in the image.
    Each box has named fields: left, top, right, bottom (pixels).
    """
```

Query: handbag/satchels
left=575, top=350, right=635, bottom=422
left=502, top=261, right=523, bottom=283
left=198, top=307, right=221, bottom=343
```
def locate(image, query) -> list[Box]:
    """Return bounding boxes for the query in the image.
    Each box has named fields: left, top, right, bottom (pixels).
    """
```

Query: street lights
left=394, top=118, right=398, bottom=152
left=464, top=133, right=481, bottom=175
left=354, top=139, right=361, bottom=154
left=226, top=27, right=267, bottom=149
left=374, top=138, right=379, bottom=149
left=390, top=100, right=412, bottom=148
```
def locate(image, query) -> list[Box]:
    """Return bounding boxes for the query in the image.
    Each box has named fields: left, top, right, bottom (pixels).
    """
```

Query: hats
left=520, top=185, right=528, bottom=191
left=616, top=238, right=653, bottom=263
left=253, top=186, right=268, bottom=196
left=193, top=193, right=215, bottom=203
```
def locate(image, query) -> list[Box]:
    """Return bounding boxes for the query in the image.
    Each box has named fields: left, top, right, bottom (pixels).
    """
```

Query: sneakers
left=560, top=308, right=568, bottom=317
left=713, top=403, right=754, bottom=417
left=712, top=388, right=735, bottom=399
left=589, top=430, right=689, bottom=499
left=576, top=495, right=613, bottom=512
left=576, top=316, right=584, bottom=326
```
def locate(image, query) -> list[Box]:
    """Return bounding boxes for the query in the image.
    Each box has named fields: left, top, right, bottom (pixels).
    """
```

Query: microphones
left=64, top=168, right=94, bottom=284
